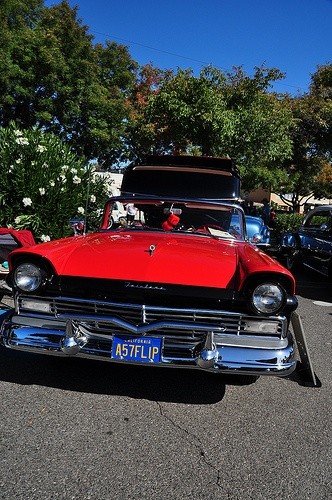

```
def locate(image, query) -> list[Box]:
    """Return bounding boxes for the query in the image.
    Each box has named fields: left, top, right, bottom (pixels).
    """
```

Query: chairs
left=147, top=212, right=221, bottom=233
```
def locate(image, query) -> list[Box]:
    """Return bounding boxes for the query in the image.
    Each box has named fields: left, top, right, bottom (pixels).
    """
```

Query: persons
left=233, top=198, right=275, bottom=224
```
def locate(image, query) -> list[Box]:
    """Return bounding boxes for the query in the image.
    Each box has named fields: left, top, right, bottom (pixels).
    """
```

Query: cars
left=229, top=214, right=270, bottom=251
left=0, top=161, right=299, bottom=377
left=282, top=205, right=332, bottom=281
